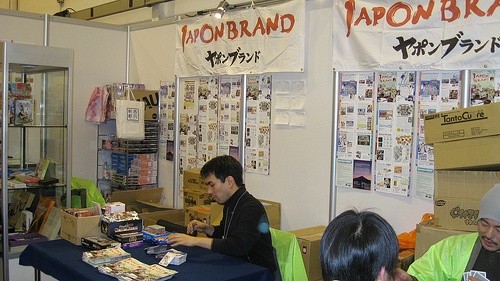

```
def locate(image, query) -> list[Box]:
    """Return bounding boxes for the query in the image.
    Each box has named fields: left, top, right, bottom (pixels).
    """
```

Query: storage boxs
left=109, top=87, right=159, bottom=120
left=60, top=167, right=281, bottom=249
left=413, top=100, right=500, bottom=263
left=287, top=224, right=327, bottom=281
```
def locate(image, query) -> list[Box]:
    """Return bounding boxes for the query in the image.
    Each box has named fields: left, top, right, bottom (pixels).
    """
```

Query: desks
left=19, top=227, right=275, bottom=281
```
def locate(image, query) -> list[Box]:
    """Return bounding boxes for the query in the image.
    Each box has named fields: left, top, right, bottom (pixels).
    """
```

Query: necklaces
left=223, top=191, right=247, bottom=239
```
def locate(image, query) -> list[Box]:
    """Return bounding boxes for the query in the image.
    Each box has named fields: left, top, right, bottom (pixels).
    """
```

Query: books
left=7, top=81, right=36, bottom=125
left=8, top=158, right=60, bottom=188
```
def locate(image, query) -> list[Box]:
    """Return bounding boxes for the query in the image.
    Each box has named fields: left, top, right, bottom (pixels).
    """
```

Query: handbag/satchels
left=114, top=87, right=145, bottom=142
left=71, top=176, right=105, bottom=209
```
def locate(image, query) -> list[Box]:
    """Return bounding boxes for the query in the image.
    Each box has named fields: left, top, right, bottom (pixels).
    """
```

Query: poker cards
left=462, top=269, right=491, bottom=281
left=157, top=248, right=188, bottom=268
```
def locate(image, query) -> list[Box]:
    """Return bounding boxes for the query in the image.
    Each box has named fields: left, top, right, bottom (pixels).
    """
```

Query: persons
left=395, top=184, right=500, bottom=281
left=319, top=209, right=398, bottom=281
left=166, top=155, right=277, bottom=272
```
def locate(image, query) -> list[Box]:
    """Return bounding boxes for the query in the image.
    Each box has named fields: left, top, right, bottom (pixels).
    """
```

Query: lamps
left=214, top=0, right=229, bottom=20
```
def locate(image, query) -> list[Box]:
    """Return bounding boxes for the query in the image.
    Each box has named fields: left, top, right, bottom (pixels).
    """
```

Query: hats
left=476, top=183, right=500, bottom=222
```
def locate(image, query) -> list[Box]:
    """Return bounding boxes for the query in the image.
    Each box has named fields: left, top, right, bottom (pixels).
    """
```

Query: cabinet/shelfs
left=0, top=41, right=75, bottom=281
left=95, top=86, right=161, bottom=190
